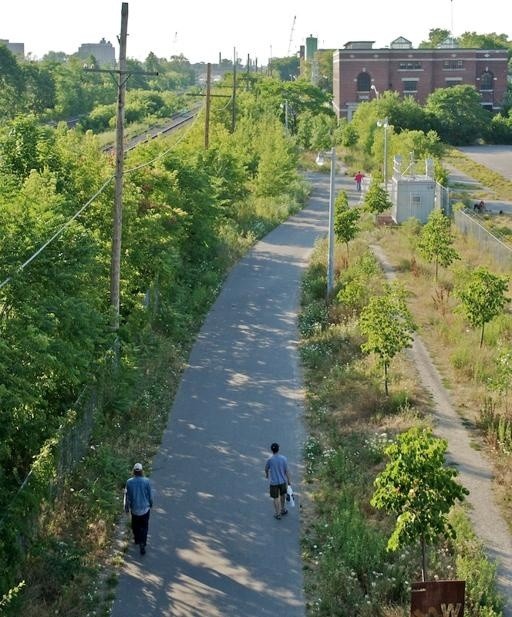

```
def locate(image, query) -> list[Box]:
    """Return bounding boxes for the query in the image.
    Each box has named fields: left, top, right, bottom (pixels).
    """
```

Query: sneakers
left=140, top=543, right=146, bottom=555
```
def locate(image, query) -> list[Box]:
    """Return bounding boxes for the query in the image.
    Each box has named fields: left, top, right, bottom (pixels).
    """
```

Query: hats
left=133, top=463, right=143, bottom=472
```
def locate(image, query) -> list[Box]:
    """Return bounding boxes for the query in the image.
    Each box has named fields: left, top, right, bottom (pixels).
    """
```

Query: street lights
left=376, top=115, right=390, bottom=195
left=315, top=145, right=340, bottom=308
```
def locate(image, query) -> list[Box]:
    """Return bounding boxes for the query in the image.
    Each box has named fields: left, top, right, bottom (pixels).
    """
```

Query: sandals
left=274, top=514, right=281, bottom=519
left=281, top=508, right=288, bottom=515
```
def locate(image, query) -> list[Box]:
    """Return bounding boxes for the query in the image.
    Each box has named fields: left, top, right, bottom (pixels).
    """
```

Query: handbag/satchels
left=287, top=485, right=295, bottom=503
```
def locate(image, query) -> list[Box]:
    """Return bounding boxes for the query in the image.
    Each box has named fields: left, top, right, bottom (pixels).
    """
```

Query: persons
left=263, top=441, right=293, bottom=521
left=353, top=170, right=364, bottom=191
left=123, top=462, right=154, bottom=556
